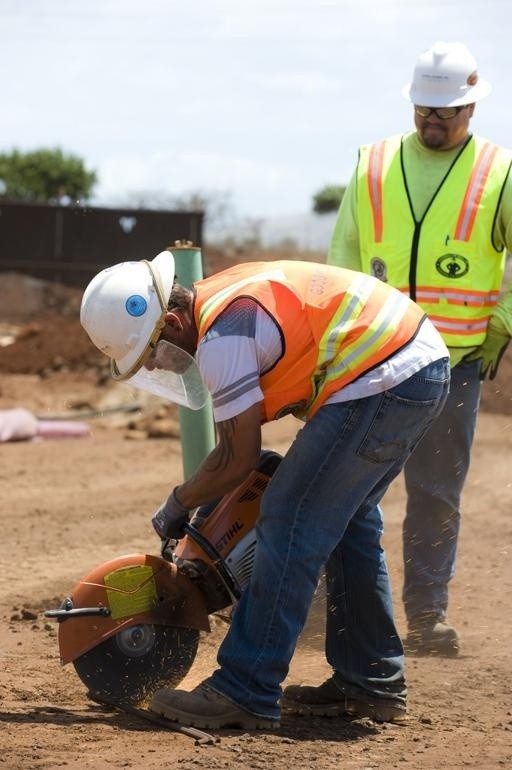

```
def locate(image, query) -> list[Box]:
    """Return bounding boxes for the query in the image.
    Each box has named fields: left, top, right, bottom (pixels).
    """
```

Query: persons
left=324, top=41, right=512, bottom=651
left=79, top=250, right=456, bottom=733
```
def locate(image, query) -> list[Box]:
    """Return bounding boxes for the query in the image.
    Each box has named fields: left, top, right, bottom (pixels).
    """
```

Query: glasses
left=415, top=103, right=471, bottom=121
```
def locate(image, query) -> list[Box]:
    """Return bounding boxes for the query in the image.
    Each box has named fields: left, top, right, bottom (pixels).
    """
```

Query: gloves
left=462, top=327, right=512, bottom=382
left=149, top=483, right=189, bottom=543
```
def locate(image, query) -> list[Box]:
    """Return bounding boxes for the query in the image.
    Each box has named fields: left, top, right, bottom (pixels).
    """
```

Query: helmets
left=79, top=249, right=214, bottom=413
left=409, top=39, right=480, bottom=110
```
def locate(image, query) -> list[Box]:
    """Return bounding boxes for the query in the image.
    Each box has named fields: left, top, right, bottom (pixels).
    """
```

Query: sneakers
left=279, top=672, right=409, bottom=723
left=405, top=610, right=462, bottom=658
left=144, top=675, right=284, bottom=735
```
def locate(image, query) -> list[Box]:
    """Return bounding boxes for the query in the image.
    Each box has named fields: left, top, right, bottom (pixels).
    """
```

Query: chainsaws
left=44, top=449, right=285, bottom=709
